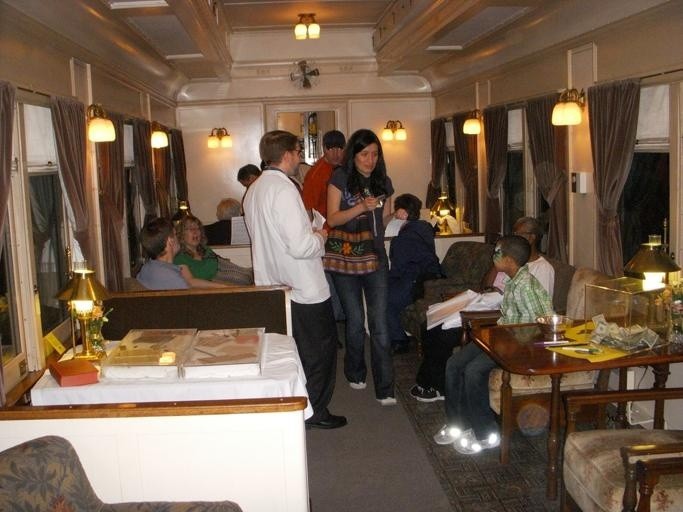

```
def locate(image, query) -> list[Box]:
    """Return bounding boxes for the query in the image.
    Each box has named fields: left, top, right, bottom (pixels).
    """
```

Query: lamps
left=551, top=88, right=582, bottom=126
left=430, top=193, right=455, bottom=232
left=172, top=201, right=191, bottom=225
left=207, top=128, right=234, bottom=149
left=294, top=13, right=320, bottom=40
left=625, top=233, right=681, bottom=330
left=463, top=109, right=480, bottom=135
left=150, top=119, right=169, bottom=150
left=381, top=119, right=407, bottom=141
left=55, top=260, right=109, bottom=360
left=86, top=102, right=117, bottom=142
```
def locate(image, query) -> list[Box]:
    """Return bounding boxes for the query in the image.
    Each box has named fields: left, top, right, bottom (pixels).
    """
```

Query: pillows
left=215, top=256, right=253, bottom=285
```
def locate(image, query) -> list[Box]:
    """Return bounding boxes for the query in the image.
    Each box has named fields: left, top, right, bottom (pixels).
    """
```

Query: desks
left=0, top=333, right=314, bottom=421
left=470, top=319, right=683, bottom=500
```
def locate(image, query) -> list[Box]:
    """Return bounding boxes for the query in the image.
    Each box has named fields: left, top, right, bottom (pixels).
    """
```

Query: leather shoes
left=306, top=414, right=347, bottom=429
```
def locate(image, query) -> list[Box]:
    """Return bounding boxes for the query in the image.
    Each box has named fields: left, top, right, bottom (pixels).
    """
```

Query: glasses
left=513, top=232, right=533, bottom=236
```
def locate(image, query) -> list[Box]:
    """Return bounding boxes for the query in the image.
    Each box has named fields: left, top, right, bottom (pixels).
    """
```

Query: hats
left=325, top=131, right=345, bottom=149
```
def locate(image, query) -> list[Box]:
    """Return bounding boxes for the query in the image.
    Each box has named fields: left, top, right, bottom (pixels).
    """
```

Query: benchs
left=99, top=286, right=292, bottom=340
left=207, top=245, right=252, bottom=268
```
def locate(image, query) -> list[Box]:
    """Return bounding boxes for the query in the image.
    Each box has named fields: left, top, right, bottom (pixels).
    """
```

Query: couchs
left=0, top=434, right=243, bottom=511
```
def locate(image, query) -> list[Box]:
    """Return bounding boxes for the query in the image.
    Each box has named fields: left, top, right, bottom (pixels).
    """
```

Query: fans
left=288, top=60, right=321, bottom=89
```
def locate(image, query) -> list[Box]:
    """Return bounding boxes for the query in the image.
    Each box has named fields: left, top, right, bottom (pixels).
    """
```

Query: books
left=181, top=327, right=267, bottom=378
left=49, top=359, right=100, bottom=388
left=102, top=328, right=199, bottom=379
left=426, top=289, right=481, bottom=331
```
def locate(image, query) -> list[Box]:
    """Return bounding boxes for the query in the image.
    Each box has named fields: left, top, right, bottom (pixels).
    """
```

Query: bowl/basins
left=535, top=316, right=572, bottom=340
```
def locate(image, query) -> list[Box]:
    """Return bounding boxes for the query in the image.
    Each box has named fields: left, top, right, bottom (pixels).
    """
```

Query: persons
left=382, top=194, right=443, bottom=357
left=321, top=128, right=398, bottom=406
left=407, top=216, right=555, bottom=403
left=432, top=234, right=555, bottom=456
left=237, top=163, right=261, bottom=187
left=302, top=130, right=347, bottom=349
left=241, top=130, right=349, bottom=429
left=135, top=217, right=192, bottom=291
left=216, top=197, right=243, bottom=222
left=173, top=215, right=254, bottom=288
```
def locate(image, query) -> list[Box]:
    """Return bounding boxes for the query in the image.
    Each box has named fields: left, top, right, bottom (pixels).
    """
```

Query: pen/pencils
left=562, top=346, right=599, bottom=353
left=534, top=340, right=569, bottom=345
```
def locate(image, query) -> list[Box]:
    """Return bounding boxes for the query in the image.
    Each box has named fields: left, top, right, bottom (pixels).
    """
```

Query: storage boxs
left=585, top=277, right=672, bottom=352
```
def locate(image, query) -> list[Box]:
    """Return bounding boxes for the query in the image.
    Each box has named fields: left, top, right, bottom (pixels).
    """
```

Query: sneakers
left=416, top=387, right=445, bottom=402
left=348, top=381, right=367, bottom=390
left=375, top=395, right=396, bottom=405
left=453, top=427, right=501, bottom=454
left=409, top=385, right=421, bottom=398
left=433, top=424, right=460, bottom=445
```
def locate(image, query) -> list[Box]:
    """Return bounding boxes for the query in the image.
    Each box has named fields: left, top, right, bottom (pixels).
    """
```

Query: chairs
left=563, top=388, right=683, bottom=511
left=636, top=458, right=683, bottom=512
